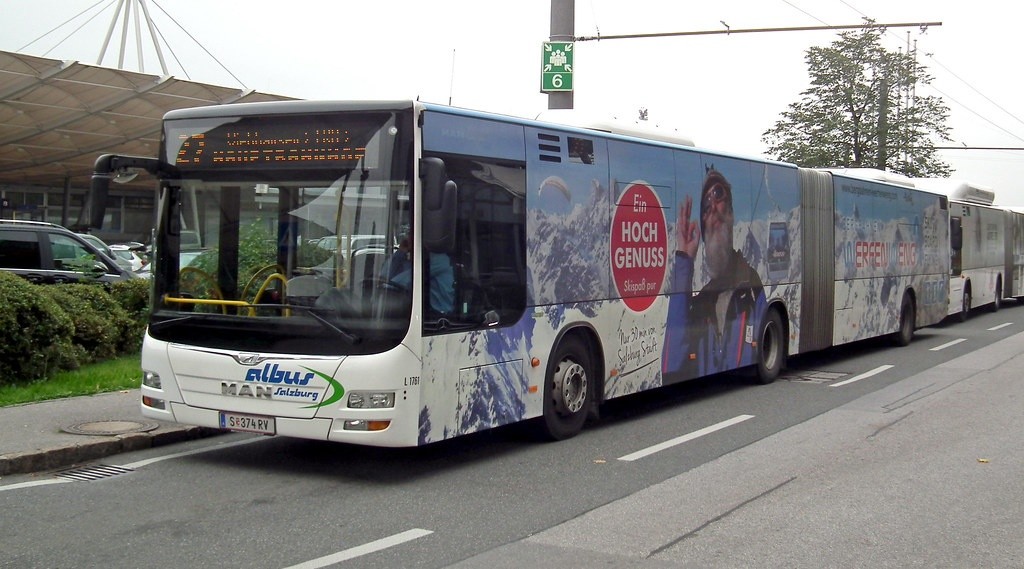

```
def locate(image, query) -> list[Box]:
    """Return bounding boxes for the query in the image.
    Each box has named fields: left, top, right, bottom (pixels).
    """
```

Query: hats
left=700, top=169, right=732, bottom=241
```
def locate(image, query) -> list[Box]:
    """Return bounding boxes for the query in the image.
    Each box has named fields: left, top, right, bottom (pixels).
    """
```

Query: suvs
left=0, top=219, right=398, bottom=297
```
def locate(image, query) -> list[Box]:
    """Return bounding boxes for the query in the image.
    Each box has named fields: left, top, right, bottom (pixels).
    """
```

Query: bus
left=78, top=98, right=949, bottom=447
left=910, top=182, right=1024, bottom=321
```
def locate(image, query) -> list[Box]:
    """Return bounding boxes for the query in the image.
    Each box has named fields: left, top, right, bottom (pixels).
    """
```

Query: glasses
left=702, top=183, right=727, bottom=206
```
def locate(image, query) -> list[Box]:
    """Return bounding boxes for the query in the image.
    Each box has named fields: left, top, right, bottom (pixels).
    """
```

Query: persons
left=660, top=162, right=769, bottom=387
left=378, top=229, right=456, bottom=314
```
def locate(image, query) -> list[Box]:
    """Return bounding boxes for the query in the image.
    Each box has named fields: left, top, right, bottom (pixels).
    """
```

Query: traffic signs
left=541, top=42, right=572, bottom=92
